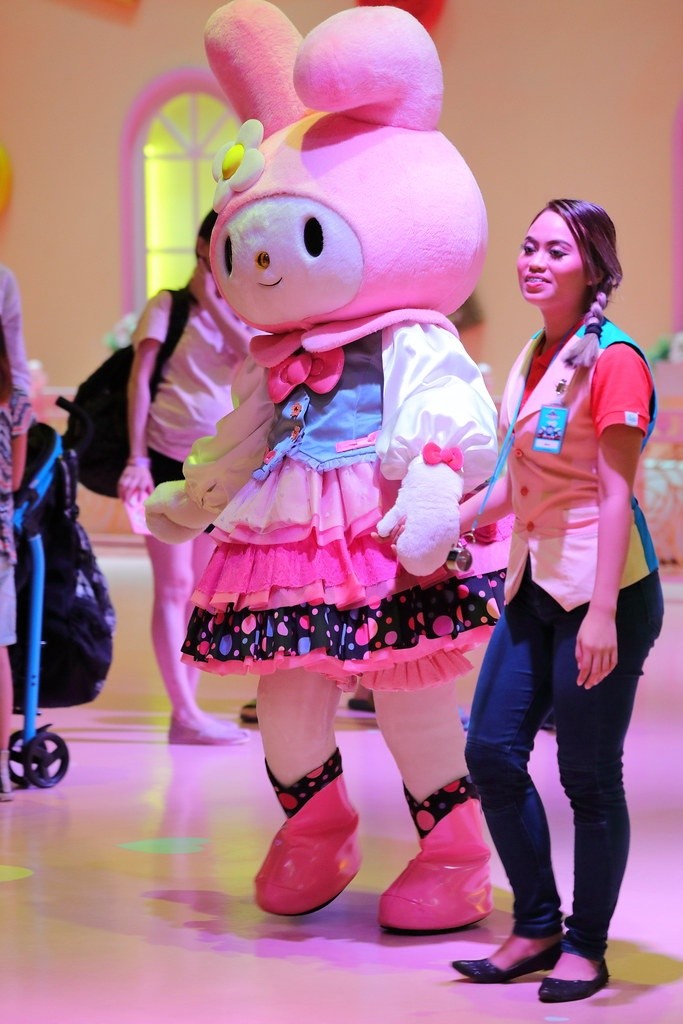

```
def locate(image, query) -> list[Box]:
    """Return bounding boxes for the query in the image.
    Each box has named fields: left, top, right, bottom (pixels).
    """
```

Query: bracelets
left=126, top=458, right=150, bottom=468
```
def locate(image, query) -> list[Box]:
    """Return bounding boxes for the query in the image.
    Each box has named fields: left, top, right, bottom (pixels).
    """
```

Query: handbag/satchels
left=6, top=450, right=116, bottom=709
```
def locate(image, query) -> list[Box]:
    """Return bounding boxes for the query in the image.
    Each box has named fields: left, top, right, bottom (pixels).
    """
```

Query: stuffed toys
left=144, top=0, right=516, bottom=936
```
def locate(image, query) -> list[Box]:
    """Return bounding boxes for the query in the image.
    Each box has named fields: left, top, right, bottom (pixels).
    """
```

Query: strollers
left=6, top=396, right=93, bottom=788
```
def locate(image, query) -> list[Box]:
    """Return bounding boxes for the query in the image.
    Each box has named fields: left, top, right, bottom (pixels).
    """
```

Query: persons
left=371, top=199, right=664, bottom=1000
left=241, top=290, right=486, bottom=725
left=118, top=207, right=483, bottom=745
left=0, top=266, right=33, bottom=801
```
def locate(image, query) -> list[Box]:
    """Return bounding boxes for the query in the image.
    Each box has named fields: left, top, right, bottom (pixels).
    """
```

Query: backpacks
left=64, top=290, right=190, bottom=498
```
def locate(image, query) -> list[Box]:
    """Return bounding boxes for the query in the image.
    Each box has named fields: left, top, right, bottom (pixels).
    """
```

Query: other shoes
left=169, top=707, right=252, bottom=746
left=452, top=936, right=566, bottom=983
left=348, top=687, right=375, bottom=712
left=539, top=958, right=608, bottom=1002
left=241, top=696, right=258, bottom=723
